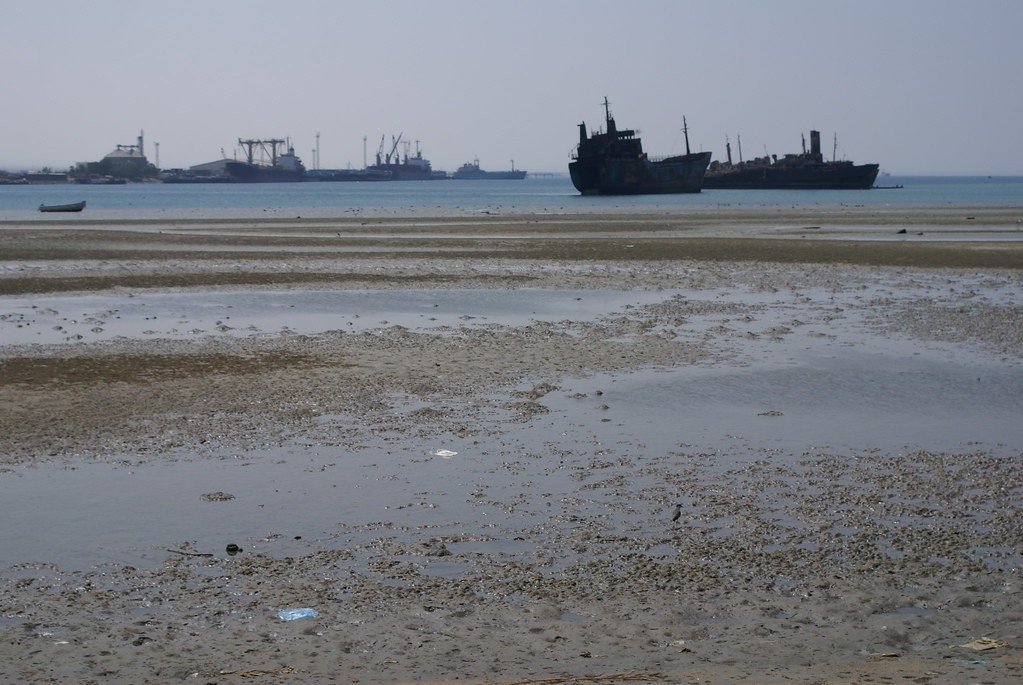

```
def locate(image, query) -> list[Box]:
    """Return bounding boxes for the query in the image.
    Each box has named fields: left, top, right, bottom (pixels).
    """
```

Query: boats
left=37, top=200, right=90, bottom=212
left=566, top=96, right=716, bottom=193
left=678, top=114, right=882, bottom=190
